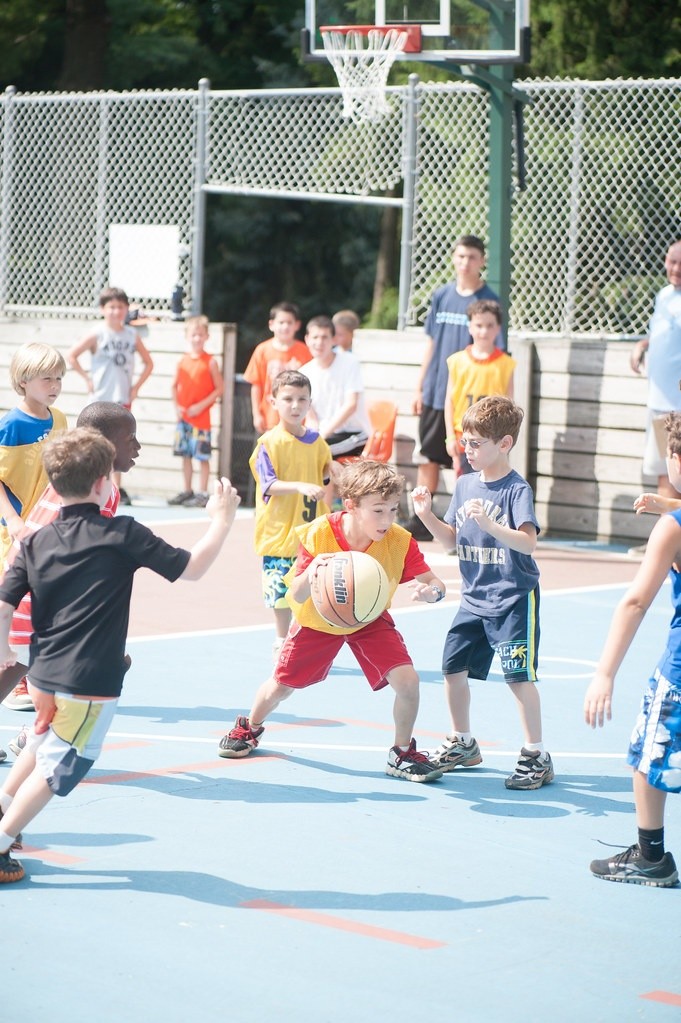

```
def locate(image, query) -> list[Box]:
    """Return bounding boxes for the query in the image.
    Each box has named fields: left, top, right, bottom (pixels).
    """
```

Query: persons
left=627, top=239, right=681, bottom=559
left=400, top=236, right=504, bottom=541
left=444, top=300, right=515, bottom=555
left=0, top=429, right=242, bottom=884
left=0, top=342, right=69, bottom=711
left=243, top=301, right=369, bottom=512
left=586, top=413, right=681, bottom=887
left=167, top=316, right=222, bottom=508
left=69, top=287, right=153, bottom=506
left=249, top=371, right=345, bottom=664
left=0, top=403, right=142, bottom=756
left=217, top=460, right=446, bottom=780
left=411, top=397, right=555, bottom=791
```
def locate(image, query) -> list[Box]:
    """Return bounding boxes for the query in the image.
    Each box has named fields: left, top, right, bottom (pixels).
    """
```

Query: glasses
left=460, top=438, right=497, bottom=449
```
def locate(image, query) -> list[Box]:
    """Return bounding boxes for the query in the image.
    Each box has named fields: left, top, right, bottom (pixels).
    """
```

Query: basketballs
left=311, top=550, right=393, bottom=630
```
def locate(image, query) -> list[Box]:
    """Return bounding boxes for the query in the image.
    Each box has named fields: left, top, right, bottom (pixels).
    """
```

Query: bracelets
left=432, top=586, right=442, bottom=602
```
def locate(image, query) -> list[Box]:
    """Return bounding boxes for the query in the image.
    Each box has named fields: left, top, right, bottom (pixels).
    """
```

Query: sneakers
left=9, top=726, right=28, bottom=756
left=386, top=737, right=443, bottom=782
left=218, top=716, right=265, bottom=758
left=166, top=490, right=193, bottom=505
left=590, top=838, right=678, bottom=887
left=182, top=492, right=211, bottom=508
left=0, top=659, right=35, bottom=711
left=0, top=804, right=23, bottom=845
left=427, top=736, right=483, bottom=771
left=0, top=850, right=24, bottom=881
left=505, top=747, right=553, bottom=791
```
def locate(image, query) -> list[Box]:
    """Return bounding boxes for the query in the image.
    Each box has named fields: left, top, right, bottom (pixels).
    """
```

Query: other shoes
left=0, top=749, right=7, bottom=762
left=399, top=517, right=435, bottom=541
left=628, top=544, right=647, bottom=556
left=119, top=488, right=131, bottom=505
